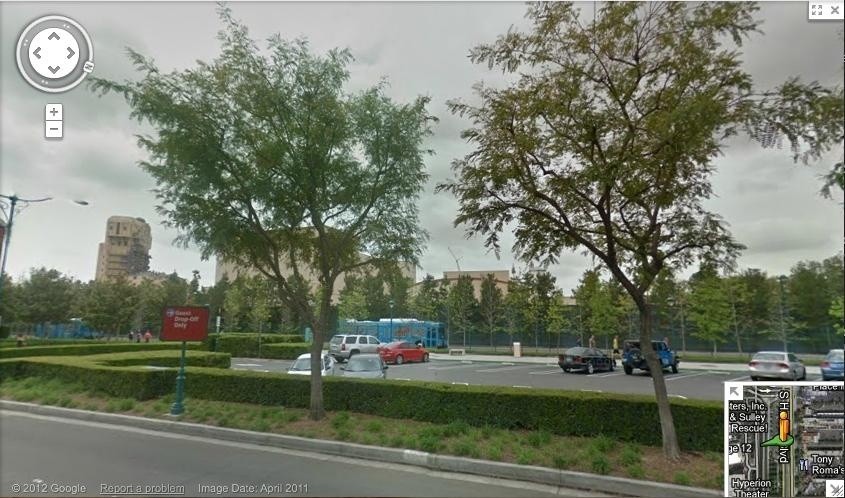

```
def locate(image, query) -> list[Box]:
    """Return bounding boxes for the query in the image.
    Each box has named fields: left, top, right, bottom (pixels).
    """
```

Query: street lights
left=2, top=195, right=87, bottom=274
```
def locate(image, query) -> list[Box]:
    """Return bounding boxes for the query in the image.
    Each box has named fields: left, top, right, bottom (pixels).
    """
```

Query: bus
left=347, top=318, right=447, bottom=349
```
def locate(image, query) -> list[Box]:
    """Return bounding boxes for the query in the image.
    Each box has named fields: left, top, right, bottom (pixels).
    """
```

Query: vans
left=329, top=334, right=386, bottom=363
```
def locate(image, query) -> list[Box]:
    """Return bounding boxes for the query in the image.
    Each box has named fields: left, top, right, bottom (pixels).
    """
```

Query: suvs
left=621, top=340, right=679, bottom=374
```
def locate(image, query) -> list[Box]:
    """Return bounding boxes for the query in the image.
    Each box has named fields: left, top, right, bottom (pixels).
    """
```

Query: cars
left=340, top=353, right=389, bottom=379
left=379, top=341, right=429, bottom=365
left=822, top=349, right=845, bottom=379
left=288, top=352, right=334, bottom=375
left=559, top=347, right=612, bottom=374
left=748, top=350, right=806, bottom=380
